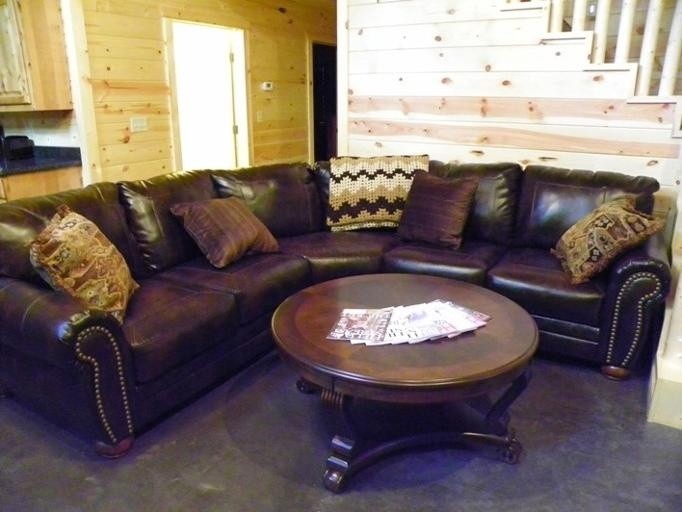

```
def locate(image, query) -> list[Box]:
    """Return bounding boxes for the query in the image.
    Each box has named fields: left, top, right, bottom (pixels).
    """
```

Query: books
left=324, top=299, right=492, bottom=347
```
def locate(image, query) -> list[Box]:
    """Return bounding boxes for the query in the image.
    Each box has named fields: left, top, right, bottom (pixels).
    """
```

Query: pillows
left=395, top=170, right=480, bottom=251
left=170, top=195, right=281, bottom=269
left=549, top=196, right=666, bottom=286
left=30, top=204, right=141, bottom=328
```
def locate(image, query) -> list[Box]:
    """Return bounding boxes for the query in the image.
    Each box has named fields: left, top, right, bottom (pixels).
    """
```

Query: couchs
left=0, top=159, right=673, bottom=457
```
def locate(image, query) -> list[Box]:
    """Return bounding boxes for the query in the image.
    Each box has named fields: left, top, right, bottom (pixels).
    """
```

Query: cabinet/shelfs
left=0, top=0, right=74, bottom=113
left=0, top=168, right=82, bottom=206
